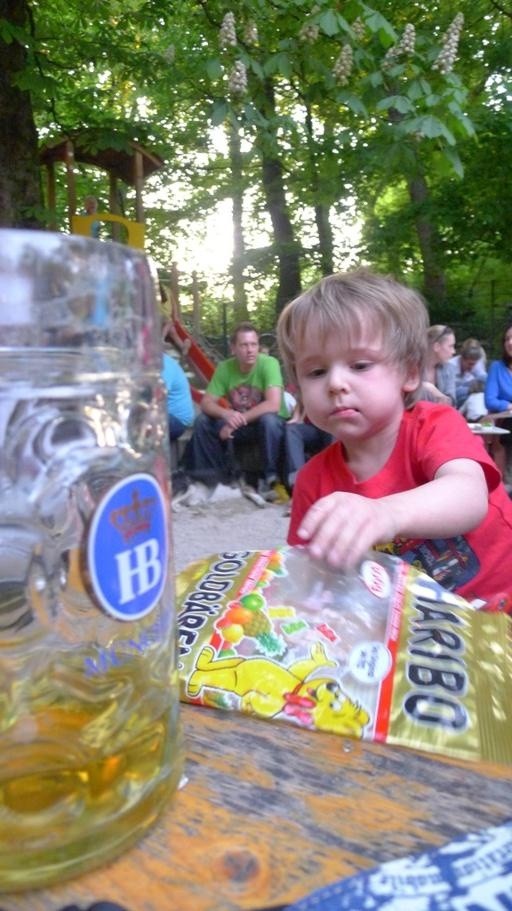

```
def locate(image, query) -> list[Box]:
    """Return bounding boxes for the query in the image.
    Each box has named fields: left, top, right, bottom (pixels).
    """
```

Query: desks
left=5, top=552, right=509, bottom=903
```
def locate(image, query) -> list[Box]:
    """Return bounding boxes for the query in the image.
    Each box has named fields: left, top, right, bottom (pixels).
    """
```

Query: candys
left=267, top=605, right=295, bottom=619
left=280, top=619, right=308, bottom=634
left=318, top=623, right=338, bottom=642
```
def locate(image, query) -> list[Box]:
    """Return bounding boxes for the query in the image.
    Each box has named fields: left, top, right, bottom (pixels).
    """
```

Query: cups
left=1, top=220, right=183, bottom=901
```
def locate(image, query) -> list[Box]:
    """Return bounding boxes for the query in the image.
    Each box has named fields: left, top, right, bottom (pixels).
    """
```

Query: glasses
left=434, top=326, right=452, bottom=343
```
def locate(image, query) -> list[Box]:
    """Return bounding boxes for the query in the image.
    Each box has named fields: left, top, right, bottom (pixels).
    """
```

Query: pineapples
left=243, top=611, right=289, bottom=657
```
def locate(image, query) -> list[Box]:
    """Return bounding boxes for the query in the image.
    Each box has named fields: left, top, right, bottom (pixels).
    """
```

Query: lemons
left=222, top=623, right=242, bottom=642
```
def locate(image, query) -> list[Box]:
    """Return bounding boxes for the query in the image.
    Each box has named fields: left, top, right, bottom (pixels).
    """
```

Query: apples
left=240, top=594, right=264, bottom=611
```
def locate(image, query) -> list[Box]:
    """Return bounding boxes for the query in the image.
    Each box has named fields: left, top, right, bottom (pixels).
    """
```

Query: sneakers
left=272, top=484, right=288, bottom=505
left=186, top=480, right=209, bottom=506
left=207, top=482, right=242, bottom=502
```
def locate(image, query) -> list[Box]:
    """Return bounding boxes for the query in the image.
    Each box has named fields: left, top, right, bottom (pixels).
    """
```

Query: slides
left=172, top=318, right=220, bottom=383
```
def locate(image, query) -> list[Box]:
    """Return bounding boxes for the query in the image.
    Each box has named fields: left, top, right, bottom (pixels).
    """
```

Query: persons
left=80, top=197, right=102, bottom=241
left=129, top=352, right=194, bottom=439
left=183, top=323, right=335, bottom=514
left=423, top=325, right=511, bottom=485
left=275, top=269, right=511, bottom=620
